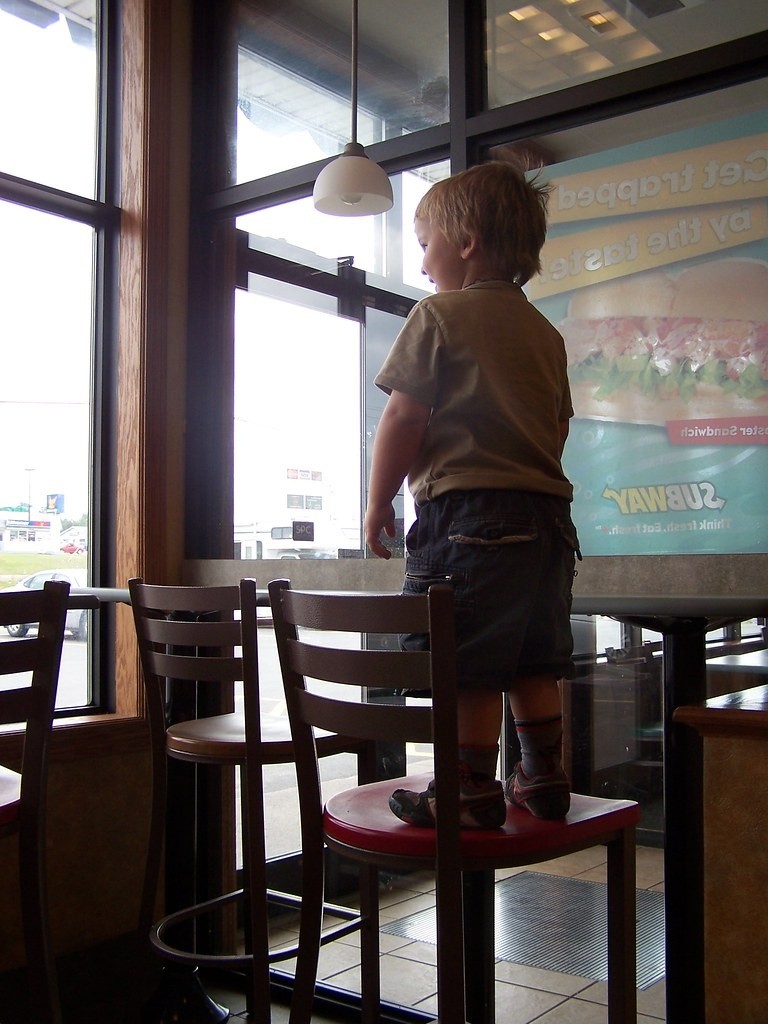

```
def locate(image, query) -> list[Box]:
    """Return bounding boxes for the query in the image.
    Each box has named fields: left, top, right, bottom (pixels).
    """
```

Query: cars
left=60, top=542, right=85, bottom=554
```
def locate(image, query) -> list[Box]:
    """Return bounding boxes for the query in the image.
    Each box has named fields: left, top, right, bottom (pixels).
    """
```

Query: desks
left=66, top=586, right=768, bottom=1024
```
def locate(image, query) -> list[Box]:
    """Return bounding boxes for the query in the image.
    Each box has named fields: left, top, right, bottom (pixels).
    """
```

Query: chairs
left=0, top=578, right=74, bottom=1024
left=127, top=575, right=642, bottom=1024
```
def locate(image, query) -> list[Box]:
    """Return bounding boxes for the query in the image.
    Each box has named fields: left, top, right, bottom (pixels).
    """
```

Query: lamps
left=311, top=0, right=394, bottom=220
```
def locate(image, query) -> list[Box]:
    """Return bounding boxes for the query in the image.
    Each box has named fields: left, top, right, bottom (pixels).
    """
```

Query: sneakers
left=389, top=773, right=506, bottom=829
left=506, top=760, right=572, bottom=821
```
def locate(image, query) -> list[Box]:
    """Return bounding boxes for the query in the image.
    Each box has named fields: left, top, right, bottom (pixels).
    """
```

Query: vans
left=278, top=553, right=317, bottom=560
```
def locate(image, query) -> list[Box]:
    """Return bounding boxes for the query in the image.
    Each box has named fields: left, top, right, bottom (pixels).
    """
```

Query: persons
left=364, top=153, right=583, bottom=832
